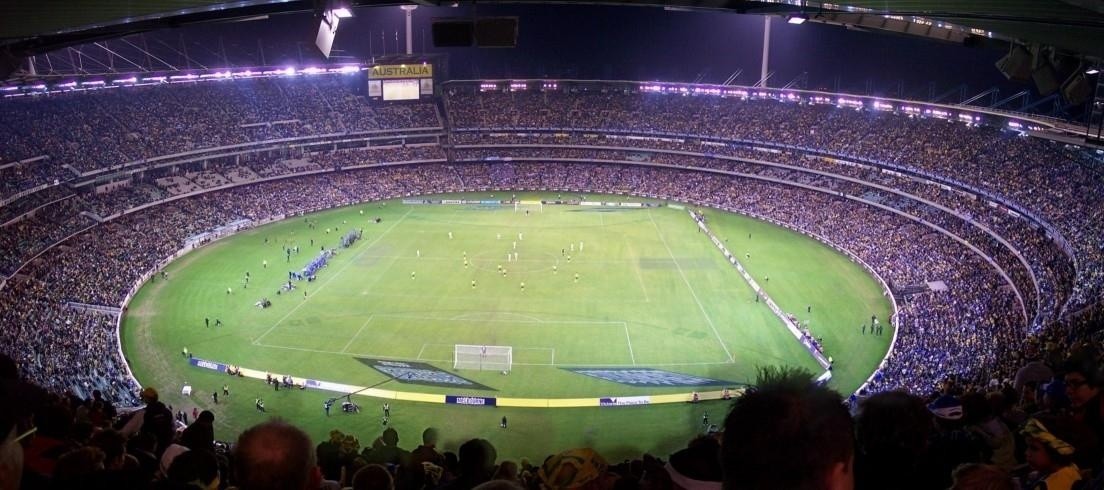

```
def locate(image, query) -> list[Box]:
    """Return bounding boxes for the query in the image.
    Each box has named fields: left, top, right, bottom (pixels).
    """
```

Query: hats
left=143, top=388, right=159, bottom=400
left=926, top=395, right=963, bottom=419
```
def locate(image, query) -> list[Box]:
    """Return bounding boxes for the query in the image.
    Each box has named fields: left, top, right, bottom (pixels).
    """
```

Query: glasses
left=1062, top=379, right=1090, bottom=389
